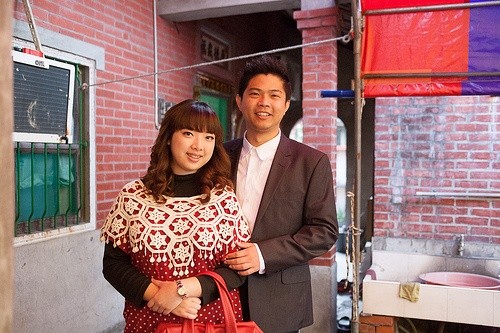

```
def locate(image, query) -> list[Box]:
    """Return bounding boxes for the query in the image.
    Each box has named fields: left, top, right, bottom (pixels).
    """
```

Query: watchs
left=177, top=280, right=189, bottom=300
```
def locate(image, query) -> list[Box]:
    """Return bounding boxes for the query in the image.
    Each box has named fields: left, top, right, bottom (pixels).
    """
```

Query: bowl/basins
left=418, top=272, right=500, bottom=290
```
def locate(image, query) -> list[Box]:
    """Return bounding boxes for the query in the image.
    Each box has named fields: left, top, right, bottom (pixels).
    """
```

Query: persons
left=99, top=99, right=254, bottom=332
left=223, top=55, right=340, bottom=333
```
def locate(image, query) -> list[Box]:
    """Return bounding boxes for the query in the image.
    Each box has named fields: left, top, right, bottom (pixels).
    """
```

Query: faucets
left=454, top=232, right=467, bottom=257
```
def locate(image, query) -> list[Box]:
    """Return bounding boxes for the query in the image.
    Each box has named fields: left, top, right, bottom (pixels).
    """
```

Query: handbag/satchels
left=155, top=269, right=263, bottom=332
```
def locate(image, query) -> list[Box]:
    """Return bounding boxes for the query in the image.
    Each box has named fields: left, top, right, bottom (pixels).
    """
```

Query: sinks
left=363, top=248, right=500, bottom=327
